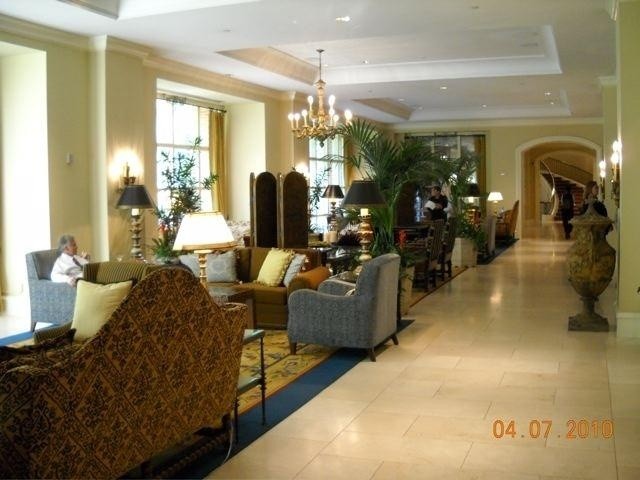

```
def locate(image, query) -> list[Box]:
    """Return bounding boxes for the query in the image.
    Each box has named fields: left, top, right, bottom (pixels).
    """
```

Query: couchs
left=0, top=266, right=248, bottom=480
left=177, top=245, right=331, bottom=330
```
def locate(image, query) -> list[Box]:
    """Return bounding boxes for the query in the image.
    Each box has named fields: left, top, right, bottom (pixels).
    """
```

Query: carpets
left=6, top=258, right=468, bottom=428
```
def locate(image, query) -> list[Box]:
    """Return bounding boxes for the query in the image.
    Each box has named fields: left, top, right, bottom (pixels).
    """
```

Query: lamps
left=598, top=160, right=606, bottom=201
left=287, top=48, right=354, bottom=147
left=172, top=213, right=237, bottom=304
left=342, top=180, right=387, bottom=277
left=609, top=140, right=621, bottom=208
left=116, top=185, right=156, bottom=264
left=320, top=183, right=345, bottom=246
left=487, top=191, right=504, bottom=223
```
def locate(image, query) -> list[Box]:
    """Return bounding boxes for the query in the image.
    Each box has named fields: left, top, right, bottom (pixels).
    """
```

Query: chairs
left=26, top=247, right=93, bottom=330
left=328, top=199, right=519, bottom=292
left=35, top=262, right=159, bottom=353
left=286, top=252, right=401, bottom=362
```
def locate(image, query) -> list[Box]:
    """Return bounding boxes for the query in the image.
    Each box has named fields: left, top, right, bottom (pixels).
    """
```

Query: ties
left=73, top=257, right=84, bottom=272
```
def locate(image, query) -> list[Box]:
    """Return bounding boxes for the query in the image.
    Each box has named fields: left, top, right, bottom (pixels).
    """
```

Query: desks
left=233, top=326, right=266, bottom=443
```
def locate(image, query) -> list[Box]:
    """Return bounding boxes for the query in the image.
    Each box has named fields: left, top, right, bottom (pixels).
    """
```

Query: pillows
left=177, top=254, right=203, bottom=278
left=282, top=252, right=306, bottom=288
left=72, top=277, right=135, bottom=340
left=0, top=328, right=79, bottom=368
left=206, top=251, right=240, bottom=283
left=253, top=248, right=295, bottom=287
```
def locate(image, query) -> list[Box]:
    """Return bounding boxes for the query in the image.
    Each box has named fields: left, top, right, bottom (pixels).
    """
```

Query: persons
left=51, top=234, right=93, bottom=287
left=581, top=181, right=613, bottom=230
left=559, top=185, right=577, bottom=240
left=426, top=186, right=449, bottom=223
